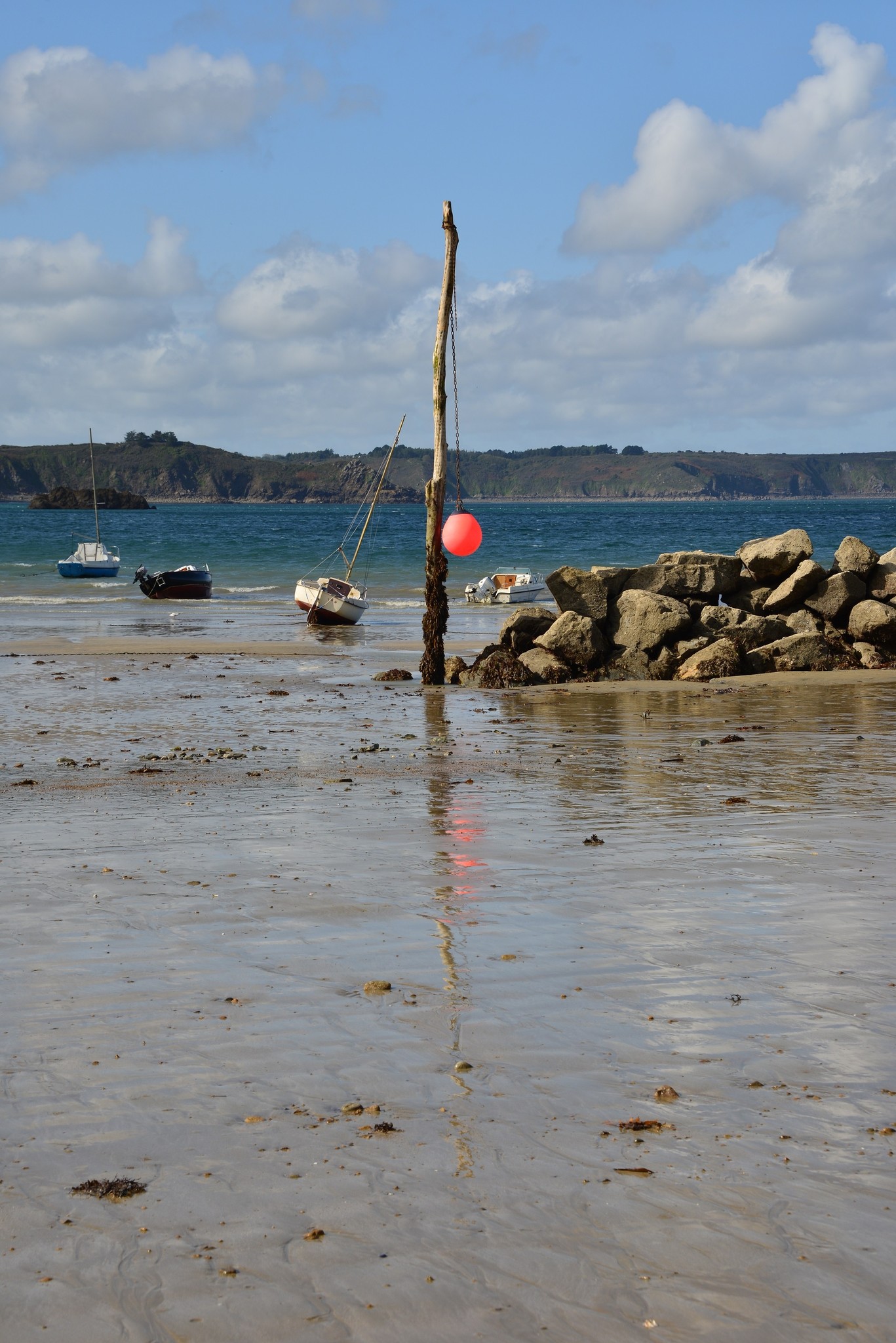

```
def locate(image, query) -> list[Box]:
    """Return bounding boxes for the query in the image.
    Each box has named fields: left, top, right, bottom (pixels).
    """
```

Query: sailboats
left=293, top=415, right=406, bottom=627
left=57, top=428, right=121, bottom=578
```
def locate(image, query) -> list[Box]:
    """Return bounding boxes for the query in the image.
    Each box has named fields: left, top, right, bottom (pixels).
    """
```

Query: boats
left=465, top=566, right=544, bottom=603
left=132, top=564, right=212, bottom=600
